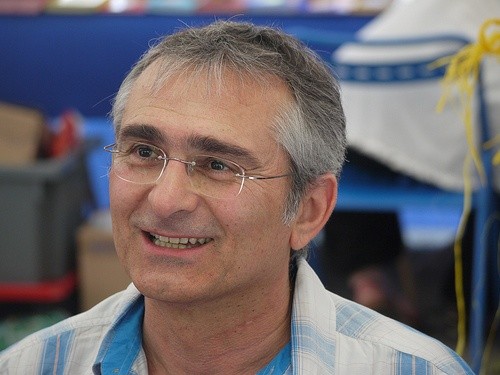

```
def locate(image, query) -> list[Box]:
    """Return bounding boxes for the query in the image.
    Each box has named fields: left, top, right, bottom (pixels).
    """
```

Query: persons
left=0, top=13, right=476, bottom=375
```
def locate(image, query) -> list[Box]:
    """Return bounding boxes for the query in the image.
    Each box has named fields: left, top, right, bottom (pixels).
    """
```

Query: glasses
left=104, top=142, right=296, bottom=200
